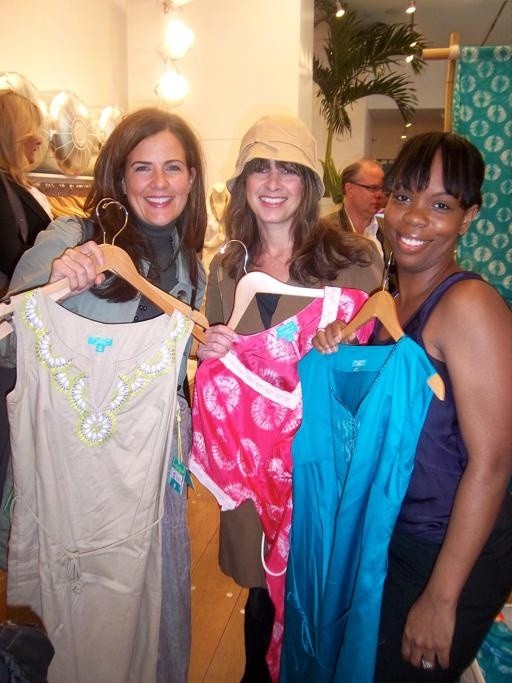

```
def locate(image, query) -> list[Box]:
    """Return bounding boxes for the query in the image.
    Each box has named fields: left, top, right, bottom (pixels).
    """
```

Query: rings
left=420, top=659, right=433, bottom=670
left=317, top=328, right=325, bottom=333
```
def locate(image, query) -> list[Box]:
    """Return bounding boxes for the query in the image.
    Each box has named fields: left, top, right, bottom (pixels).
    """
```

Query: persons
left=309, top=130, right=512, bottom=683
left=0, top=88, right=51, bottom=504
left=1, top=109, right=205, bottom=682
left=207, top=112, right=384, bottom=682
left=313, top=157, right=395, bottom=293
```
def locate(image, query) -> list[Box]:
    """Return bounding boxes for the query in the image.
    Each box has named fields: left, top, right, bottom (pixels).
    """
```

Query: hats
left=226, top=114, right=326, bottom=199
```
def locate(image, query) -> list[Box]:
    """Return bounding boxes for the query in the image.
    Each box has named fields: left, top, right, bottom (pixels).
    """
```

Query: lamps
left=405, top=0, right=416, bottom=13
left=334, top=0, right=345, bottom=18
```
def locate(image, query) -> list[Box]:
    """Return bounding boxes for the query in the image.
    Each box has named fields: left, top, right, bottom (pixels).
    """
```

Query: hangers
left=343, top=249, right=445, bottom=403
left=222, top=239, right=326, bottom=331
left=0, top=183, right=209, bottom=347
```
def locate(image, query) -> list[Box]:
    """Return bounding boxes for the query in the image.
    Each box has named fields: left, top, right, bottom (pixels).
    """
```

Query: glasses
left=352, top=180, right=384, bottom=192
